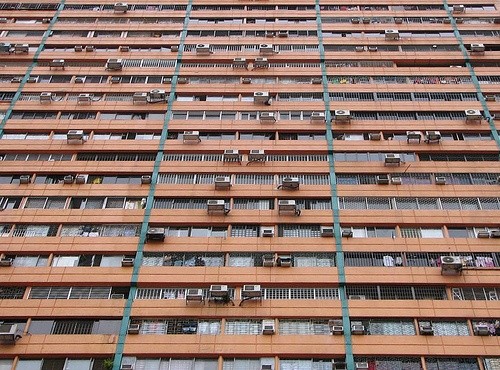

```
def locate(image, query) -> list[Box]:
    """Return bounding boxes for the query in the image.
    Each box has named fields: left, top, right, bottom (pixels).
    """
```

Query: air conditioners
left=478, top=231, right=500, bottom=238
left=128, top=324, right=139, bottom=334
left=262, top=324, right=274, bottom=334
left=111, top=294, right=124, bottom=299
left=0, top=324, right=18, bottom=341
left=420, top=325, right=435, bottom=336
left=0, top=0, right=500, bottom=191
left=333, top=362, right=368, bottom=370
left=263, top=258, right=291, bottom=266
left=207, top=200, right=224, bottom=210
left=186, top=284, right=261, bottom=300
left=322, top=229, right=352, bottom=236
left=0, top=258, right=12, bottom=266
left=440, top=256, right=462, bottom=270
left=147, top=228, right=164, bottom=239
left=278, top=200, right=296, bottom=211
left=473, top=324, right=489, bottom=336
left=121, top=258, right=134, bottom=266
left=120, top=364, right=133, bottom=370
left=263, top=229, right=274, bottom=236
left=262, top=364, right=272, bottom=370
left=331, top=325, right=364, bottom=334
